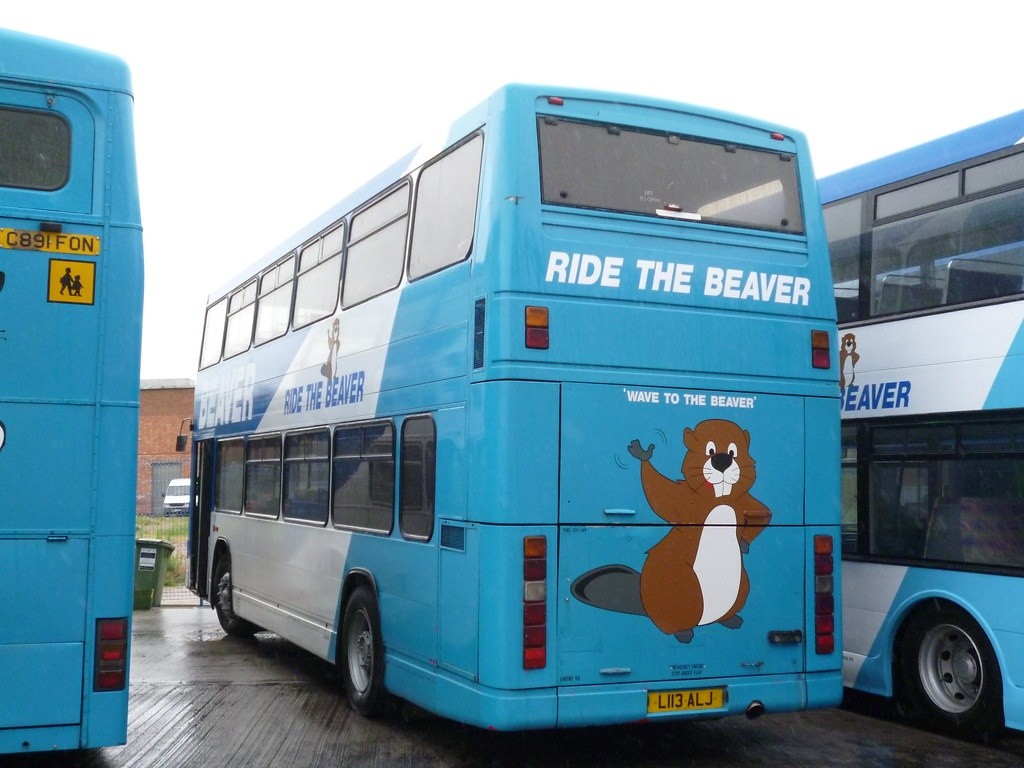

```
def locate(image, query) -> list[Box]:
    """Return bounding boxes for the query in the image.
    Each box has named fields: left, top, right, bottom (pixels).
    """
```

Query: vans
left=160, top=478, right=198, bottom=517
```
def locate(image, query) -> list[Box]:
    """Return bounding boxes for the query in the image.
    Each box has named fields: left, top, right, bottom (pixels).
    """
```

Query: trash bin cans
left=132, top=538, right=175, bottom=609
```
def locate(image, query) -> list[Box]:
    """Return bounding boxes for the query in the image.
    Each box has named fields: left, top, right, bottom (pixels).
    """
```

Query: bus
left=700, top=109, right=1024, bottom=742
left=189, top=94, right=848, bottom=734
left=1, top=30, right=143, bottom=755
